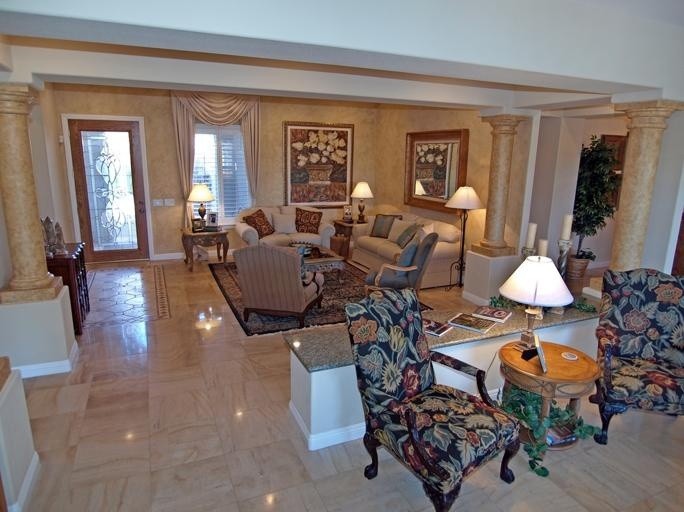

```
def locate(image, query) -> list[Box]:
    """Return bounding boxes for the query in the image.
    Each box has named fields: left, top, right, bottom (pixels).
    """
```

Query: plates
left=47, top=242, right=91, bottom=336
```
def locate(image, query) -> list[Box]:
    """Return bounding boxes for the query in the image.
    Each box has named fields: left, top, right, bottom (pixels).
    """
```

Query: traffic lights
left=297, top=246, right=308, bottom=279
left=366, top=213, right=436, bottom=248
left=269, top=213, right=298, bottom=234
left=243, top=210, right=274, bottom=238
left=295, top=208, right=323, bottom=233
left=396, top=235, right=419, bottom=276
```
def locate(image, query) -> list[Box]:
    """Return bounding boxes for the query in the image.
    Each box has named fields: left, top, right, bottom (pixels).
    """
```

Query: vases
left=292, top=130, right=347, bottom=169
left=416, top=143, right=447, bottom=165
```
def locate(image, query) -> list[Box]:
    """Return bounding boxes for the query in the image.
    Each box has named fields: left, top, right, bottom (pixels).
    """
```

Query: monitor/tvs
left=447, top=312, right=497, bottom=335
left=471, top=305, right=514, bottom=324
left=546, top=424, right=578, bottom=447
left=422, top=318, right=454, bottom=338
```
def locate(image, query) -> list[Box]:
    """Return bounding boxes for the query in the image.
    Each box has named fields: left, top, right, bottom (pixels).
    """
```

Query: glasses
left=444, top=186, right=486, bottom=290
left=188, top=185, right=215, bottom=219
left=351, top=181, right=375, bottom=224
left=415, top=181, right=427, bottom=195
left=498, top=255, right=574, bottom=353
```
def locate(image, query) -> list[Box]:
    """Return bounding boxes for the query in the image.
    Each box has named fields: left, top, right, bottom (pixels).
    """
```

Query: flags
left=305, top=164, right=333, bottom=185
left=416, top=165, right=437, bottom=179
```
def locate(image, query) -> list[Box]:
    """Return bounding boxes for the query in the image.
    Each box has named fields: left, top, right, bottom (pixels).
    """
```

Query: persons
left=211, top=215, right=215, bottom=223
left=344, top=207, right=351, bottom=215
left=423, top=321, right=447, bottom=333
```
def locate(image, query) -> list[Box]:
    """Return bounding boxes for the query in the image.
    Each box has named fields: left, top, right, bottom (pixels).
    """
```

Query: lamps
left=233, top=245, right=324, bottom=328
left=593, top=266, right=684, bottom=444
left=342, top=287, right=522, bottom=512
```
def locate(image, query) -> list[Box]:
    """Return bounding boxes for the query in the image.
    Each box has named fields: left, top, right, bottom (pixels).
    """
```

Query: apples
left=524, top=221, right=538, bottom=249
left=537, top=237, right=548, bottom=257
left=561, top=210, right=574, bottom=240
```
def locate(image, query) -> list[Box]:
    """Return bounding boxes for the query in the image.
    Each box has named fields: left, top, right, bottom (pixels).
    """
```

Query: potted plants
left=343, top=205, right=352, bottom=220
left=207, top=211, right=218, bottom=227
left=604, top=174, right=622, bottom=211
left=282, top=120, right=354, bottom=210
left=403, top=129, right=470, bottom=216
left=192, top=218, right=204, bottom=233
left=601, top=134, right=628, bottom=170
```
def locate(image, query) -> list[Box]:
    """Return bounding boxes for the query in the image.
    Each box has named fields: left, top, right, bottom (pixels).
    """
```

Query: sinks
left=235, top=205, right=336, bottom=247
left=347, top=208, right=466, bottom=295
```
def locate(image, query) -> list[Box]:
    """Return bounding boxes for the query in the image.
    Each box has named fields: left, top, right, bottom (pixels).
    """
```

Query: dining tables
left=567, top=132, right=624, bottom=280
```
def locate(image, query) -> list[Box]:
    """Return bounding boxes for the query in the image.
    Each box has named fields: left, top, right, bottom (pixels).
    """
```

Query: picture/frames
left=302, top=248, right=346, bottom=288
left=330, top=220, right=372, bottom=258
left=180, top=226, right=229, bottom=271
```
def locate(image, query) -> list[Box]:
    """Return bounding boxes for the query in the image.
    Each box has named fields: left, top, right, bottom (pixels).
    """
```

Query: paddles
left=207, top=261, right=435, bottom=337
